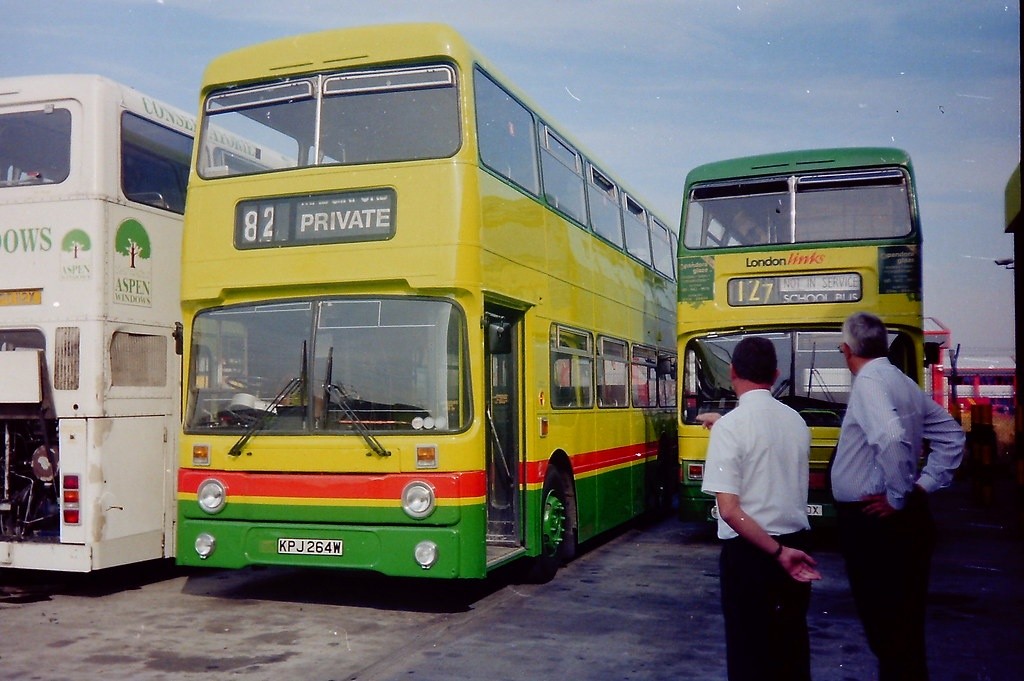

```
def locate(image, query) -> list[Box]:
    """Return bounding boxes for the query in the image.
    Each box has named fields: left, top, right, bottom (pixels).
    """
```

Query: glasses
left=838, top=343, right=848, bottom=354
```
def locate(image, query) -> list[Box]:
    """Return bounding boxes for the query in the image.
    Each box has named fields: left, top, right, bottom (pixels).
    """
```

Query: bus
left=172, top=20, right=681, bottom=581
left=0, top=75, right=299, bottom=575
left=674, top=147, right=924, bottom=526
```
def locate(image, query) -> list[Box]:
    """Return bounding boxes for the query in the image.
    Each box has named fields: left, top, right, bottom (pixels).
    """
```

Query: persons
left=830, top=311, right=967, bottom=681
left=695, top=335, right=823, bottom=681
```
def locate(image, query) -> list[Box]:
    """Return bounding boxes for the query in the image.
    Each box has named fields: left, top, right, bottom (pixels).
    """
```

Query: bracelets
left=771, top=543, right=783, bottom=560
